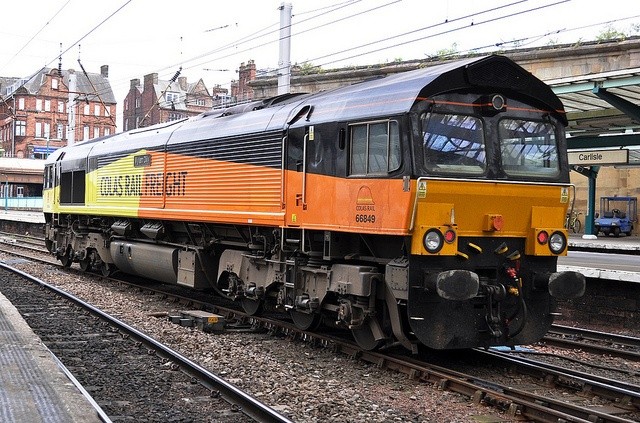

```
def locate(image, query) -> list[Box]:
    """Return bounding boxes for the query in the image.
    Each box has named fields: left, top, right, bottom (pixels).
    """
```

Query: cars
left=593, top=194, right=637, bottom=237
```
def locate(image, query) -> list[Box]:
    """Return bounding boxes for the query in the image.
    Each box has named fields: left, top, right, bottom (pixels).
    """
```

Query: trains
left=43, top=54, right=586, bottom=351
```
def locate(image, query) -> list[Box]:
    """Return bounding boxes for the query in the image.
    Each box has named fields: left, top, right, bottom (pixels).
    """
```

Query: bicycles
left=565, top=211, right=582, bottom=233
